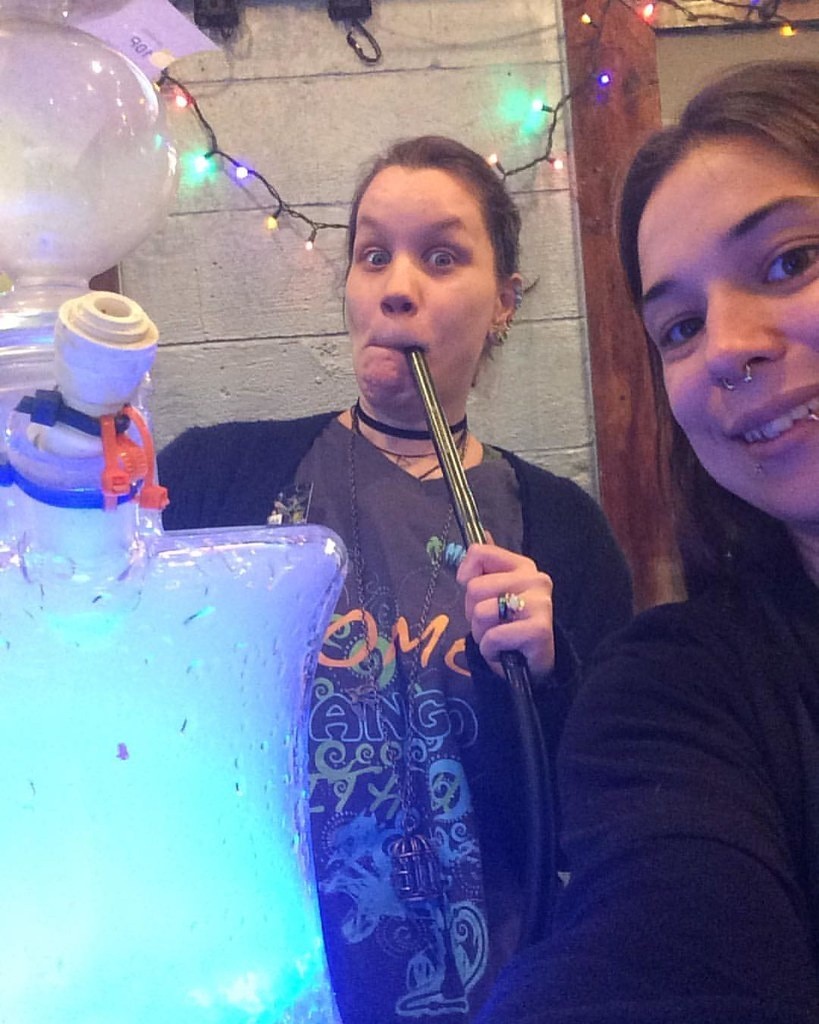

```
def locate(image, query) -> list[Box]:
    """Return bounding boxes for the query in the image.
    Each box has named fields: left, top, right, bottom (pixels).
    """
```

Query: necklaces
left=346, top=402, right=467, bottom=904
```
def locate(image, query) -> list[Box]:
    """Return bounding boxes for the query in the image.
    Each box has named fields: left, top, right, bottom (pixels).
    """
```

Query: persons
left=152, top=134, right=635, bottom=1024
left=471, top=63, right=819, bottom=1024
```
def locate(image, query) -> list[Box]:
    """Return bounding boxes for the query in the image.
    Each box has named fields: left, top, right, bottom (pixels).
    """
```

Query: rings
left=498, top=595, right=506, bottom=619
left=505, top=592, right=524, bottom=621
